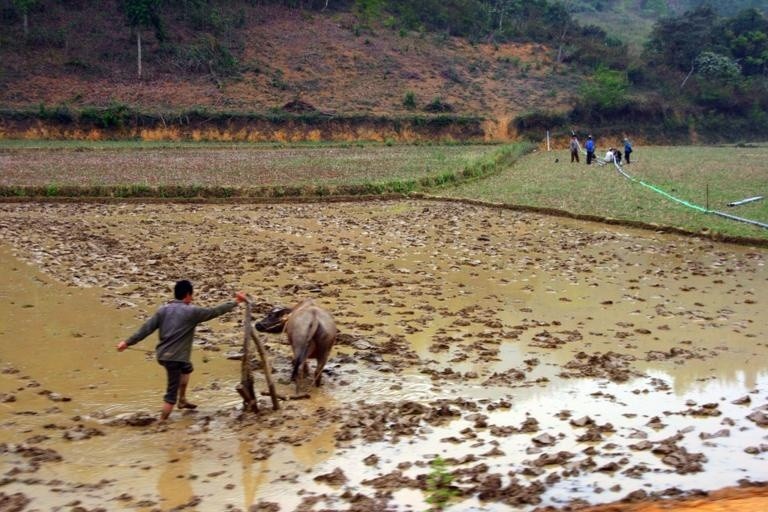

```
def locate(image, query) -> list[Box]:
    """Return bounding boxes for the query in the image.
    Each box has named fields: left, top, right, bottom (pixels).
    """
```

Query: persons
left=118, top=280, right=245, bottom=427
left=584, top=135, right=595, bottom=166
left=612, top=148, right=621, bottom=163
left=623, top=138, right=632, bottom=164
left=570, top=135, right=580, bottom=163
left=603, top=148, right=614, bottom=162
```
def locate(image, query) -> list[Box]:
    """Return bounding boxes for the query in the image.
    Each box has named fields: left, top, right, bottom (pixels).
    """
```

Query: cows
left=255, top=298, right=338, bottom=387
